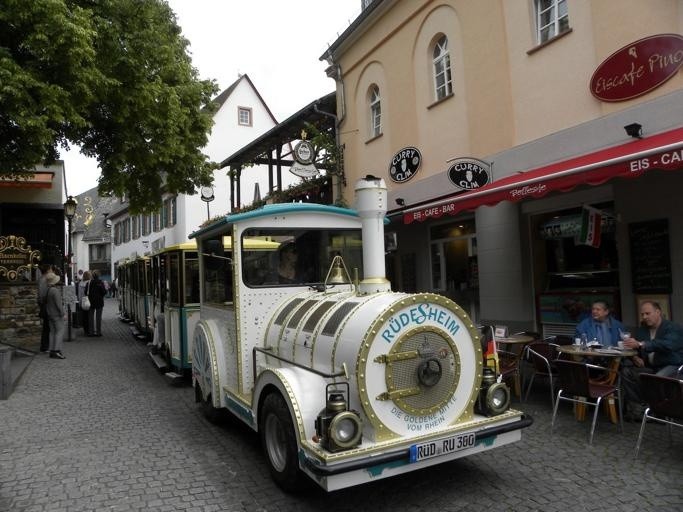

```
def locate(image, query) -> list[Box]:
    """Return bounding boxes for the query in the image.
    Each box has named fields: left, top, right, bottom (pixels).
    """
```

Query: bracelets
left=638, top=341, right=643, bottom=349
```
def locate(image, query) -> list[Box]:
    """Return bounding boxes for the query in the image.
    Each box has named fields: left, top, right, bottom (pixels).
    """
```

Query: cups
left=575, top=337, right=581, bottom=345
left=617, top=340, right=623, bottom=347
left=620, top=331, right=631, bottom=340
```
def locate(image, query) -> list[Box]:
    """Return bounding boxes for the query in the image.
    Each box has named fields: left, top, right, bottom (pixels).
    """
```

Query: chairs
left=476, top=322, right=683, bottom=459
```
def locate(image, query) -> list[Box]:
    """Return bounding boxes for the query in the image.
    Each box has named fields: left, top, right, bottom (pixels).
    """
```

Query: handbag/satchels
left=82, top=296, right=91, bottom=310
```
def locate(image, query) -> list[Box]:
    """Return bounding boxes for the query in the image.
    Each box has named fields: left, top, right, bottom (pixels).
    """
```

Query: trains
left=113, top=172, right=533, bottom=495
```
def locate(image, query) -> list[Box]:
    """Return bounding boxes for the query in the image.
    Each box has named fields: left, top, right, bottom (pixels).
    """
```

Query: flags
left=577, top=204, right=604, bottom=249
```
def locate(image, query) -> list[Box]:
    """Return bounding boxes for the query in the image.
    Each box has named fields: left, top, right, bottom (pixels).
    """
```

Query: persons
left=264, top=238, right=305, bottom=284
left=574, top=301, right=630, bottom=385
left=620, top=300, right=682, bottom=422
left=46, top=272, right=69, bottom=359
left=37, top=263, right=59, bottom=353
left=72, top=269, right=118, bottom=338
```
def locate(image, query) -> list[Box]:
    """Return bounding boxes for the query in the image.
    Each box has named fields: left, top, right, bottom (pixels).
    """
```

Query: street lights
left=62, top=194, right=76, bottom=343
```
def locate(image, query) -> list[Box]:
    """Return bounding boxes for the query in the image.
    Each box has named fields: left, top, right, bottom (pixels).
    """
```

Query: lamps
left=623, top=122, right=643, bottom=140
left=395, top=198, right=404, bottom=205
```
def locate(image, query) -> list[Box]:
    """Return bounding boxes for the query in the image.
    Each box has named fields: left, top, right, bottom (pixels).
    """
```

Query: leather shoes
left=88, top=334, right=101, bottom=337
left=40, top=350, right=65, bottom=359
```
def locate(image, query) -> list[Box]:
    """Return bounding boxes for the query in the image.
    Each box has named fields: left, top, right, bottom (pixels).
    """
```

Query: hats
left=46, top=273, right=60, bottom=285
